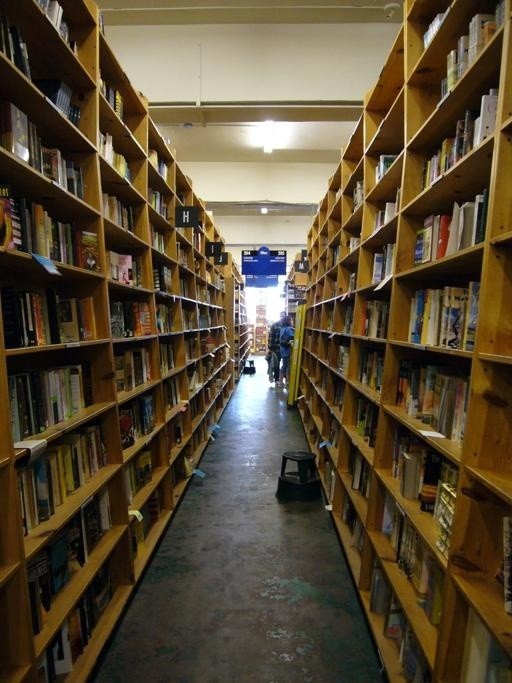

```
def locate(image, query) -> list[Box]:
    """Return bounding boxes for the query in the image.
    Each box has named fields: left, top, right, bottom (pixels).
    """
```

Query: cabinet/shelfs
left=1, top=4, right=235, bottom=681
left=285, top=284, right=306, bottom=316
left=296, top=4, right=510, bottom=672
left=247, top=303, right=269, bottom=355
left=224, top=252, right=251, bottom=384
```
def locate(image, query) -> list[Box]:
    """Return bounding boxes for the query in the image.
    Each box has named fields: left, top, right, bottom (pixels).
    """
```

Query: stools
left=243, top=359, right=255, bottom=374
left=276, top=449, right=322, bottom=503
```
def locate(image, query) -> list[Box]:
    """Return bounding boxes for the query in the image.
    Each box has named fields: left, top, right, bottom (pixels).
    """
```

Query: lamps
left=264, top=120, right=272, bottom=154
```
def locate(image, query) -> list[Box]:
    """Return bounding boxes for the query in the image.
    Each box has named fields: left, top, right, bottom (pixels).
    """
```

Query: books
left=0, top=1, right=512, bottom=683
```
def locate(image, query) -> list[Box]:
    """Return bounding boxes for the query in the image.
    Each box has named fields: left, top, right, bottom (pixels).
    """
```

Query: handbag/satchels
left=279, top=329, right=291, bottom=347
